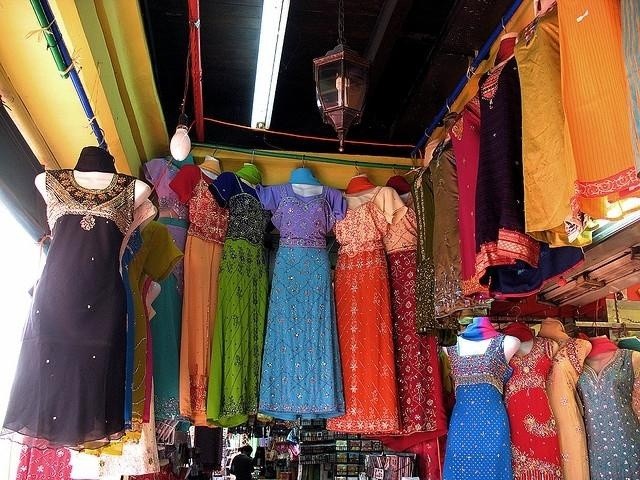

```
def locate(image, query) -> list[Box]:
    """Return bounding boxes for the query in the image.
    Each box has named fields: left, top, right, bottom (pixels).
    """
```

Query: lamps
left=170, top=45, right=192, bottom=161
left=312, top=0, right=370, bottom=152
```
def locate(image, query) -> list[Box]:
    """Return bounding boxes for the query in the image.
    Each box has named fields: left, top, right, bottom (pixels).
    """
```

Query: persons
left=582, top=335, right=639, bottom=480
left=442, top=317, right=520, bottom=480
left=535, top=317, right=592, bottom=480
left=139, top=130, right=199, bottom=436
left=0, top=145, right=152, bottom=452
left=326, top=173, right=405, bottom=436
left=230, top=446, right=254, bottom=480
left=169, top=156, right=229, bottom=426
left=500, top=321, right=559, bottom=479
left=256, top=167, right=347, bottom=421
left=380, top=175, right=447, bottom=449
left=210, top=165, right=272, bottom=426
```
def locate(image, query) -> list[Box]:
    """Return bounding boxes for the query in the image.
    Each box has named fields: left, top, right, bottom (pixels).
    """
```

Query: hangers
left=297, top=153, right=311, bottom=171
left=387, top=162, right=401, bottom=179
left=349, top=162, right=368, bottom=176
left=619, top=322, right=629, bottom=338
left=510, top=306, right=523, bottom=322
left=502, top=18, right=508, bottom=34
left=201, top=145, right=225, bottom=163
left=445, top=100, right=451, bottom=115
left=88, top=126, right=119, bottom=166
left=243, top=151, right=262, bottom=168
left=423, top=128, right=432, bottom=142
left=591, top=321, right=602, bottom=338
left=466, top=66, right=476, bottom=81
